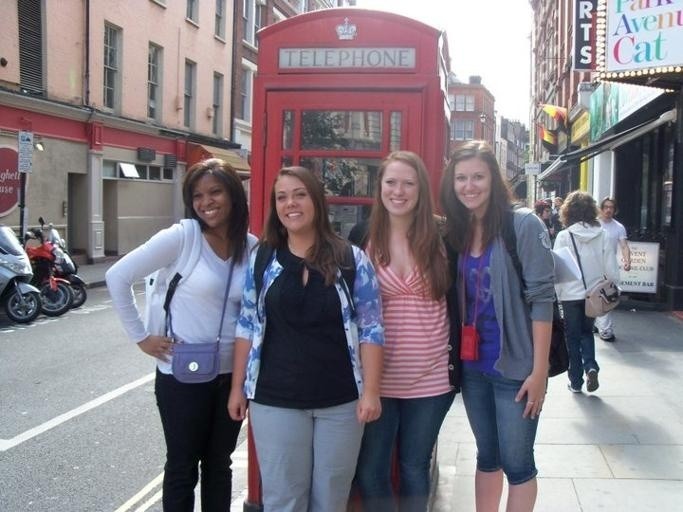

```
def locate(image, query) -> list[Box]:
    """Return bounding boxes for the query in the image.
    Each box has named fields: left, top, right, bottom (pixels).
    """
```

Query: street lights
left=479, top=113, right=486, bottom=140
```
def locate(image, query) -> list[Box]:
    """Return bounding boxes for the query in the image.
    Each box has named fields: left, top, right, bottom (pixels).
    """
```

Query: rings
left=527, top=400, right=534, bottom=404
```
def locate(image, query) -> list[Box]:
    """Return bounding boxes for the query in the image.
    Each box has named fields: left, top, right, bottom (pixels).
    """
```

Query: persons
left=437, top=138, right=558, bottom=512
left=227, top=165, right=387, bottom=511
left=343, top=148, right=458, bottom=512
left=101, top=157, right=262, bottom=512
left=528, top=188, right=633, bottom=394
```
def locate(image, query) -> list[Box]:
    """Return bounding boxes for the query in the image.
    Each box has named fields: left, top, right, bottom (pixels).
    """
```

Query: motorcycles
left=0, top=216, right=88, bottom=325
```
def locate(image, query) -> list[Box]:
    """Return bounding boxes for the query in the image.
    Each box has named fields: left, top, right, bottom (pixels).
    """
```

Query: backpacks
left=502, top=201, right=571, bottom=378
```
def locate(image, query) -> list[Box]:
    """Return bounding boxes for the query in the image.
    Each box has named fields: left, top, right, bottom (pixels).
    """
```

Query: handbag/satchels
left=171, top=340, right=222, bottom=385
left=584, top=277, right=622, bottom=319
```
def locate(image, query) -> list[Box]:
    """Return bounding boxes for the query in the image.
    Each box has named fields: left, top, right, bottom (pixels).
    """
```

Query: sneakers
left=567, top=382, right=584, bottom=393
left=592, top=326, right=616, bottom=341
left=587, top=368, right=599, bottom=392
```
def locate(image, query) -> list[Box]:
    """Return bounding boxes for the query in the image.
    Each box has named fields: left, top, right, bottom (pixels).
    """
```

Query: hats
left=534, top=197, right=553, bottom=208
